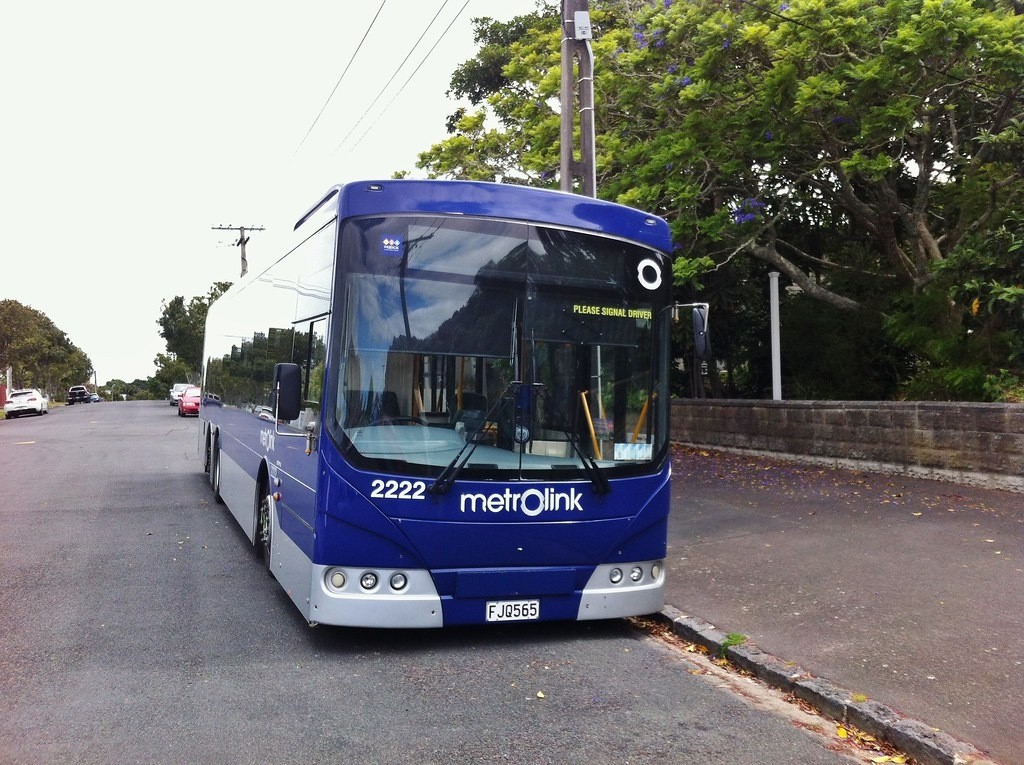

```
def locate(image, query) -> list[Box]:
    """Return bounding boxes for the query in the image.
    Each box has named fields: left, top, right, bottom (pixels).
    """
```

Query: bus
left=198, top=179, right=713, bottom=628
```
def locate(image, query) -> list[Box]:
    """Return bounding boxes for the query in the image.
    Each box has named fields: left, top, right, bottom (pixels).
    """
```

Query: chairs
left=342, top=389, right=402, bottom=430
left=451, top=392, right=487, bottom=429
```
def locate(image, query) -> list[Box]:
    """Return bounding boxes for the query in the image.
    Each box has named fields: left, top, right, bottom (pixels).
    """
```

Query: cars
left=68, top=385, right=91, bottom=405
left=91, top=393, right=99, bottom=402
left=3, top=389, right=48, bottom=420
left=177, top=387, right=201, bottom=417
left=169, top=384, right=196, bottom=406
